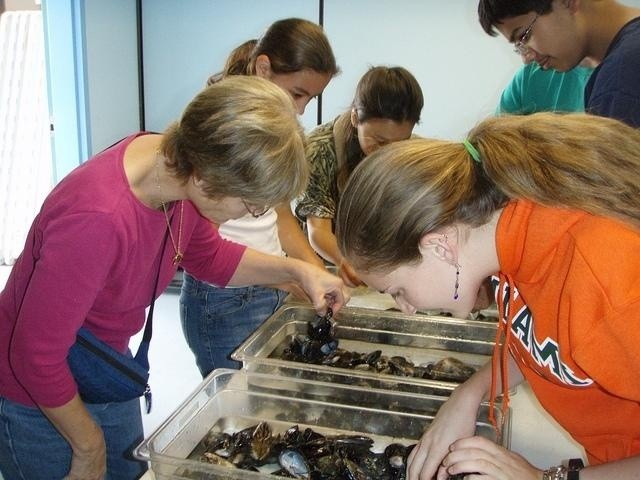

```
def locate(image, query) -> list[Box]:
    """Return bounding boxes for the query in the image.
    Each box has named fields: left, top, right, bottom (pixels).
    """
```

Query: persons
left=478, top=1, right=639, bottom=128
left=178, top=17, right=343, bottom=397
left=496, top=50, right=597, bottom=117
left=293, top=63, right=426, bottom=292
left=0, top=72, right=351, bottom=479
left=324, top=111, right=640, bottom=480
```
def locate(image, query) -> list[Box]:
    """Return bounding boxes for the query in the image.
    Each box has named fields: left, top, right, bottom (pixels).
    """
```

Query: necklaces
left=152, top=145, right=187, bottom=268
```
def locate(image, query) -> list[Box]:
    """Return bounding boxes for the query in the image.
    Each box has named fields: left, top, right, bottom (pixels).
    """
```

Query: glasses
left=511, top=12, right=544, bottom=54
left=242, top=198, right=273, bottom=219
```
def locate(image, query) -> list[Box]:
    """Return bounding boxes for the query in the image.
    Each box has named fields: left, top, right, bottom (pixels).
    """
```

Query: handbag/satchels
left=67, top=327, right=153, bottom=414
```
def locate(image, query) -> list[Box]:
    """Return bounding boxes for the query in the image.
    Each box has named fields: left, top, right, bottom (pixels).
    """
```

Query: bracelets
left=566, top=457, right=585, bottom=480
left=543, top=463, right=566, bottom=480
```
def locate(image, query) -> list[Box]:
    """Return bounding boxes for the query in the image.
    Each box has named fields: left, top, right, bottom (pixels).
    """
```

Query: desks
left=139, top=271, right=589, bottom=480
